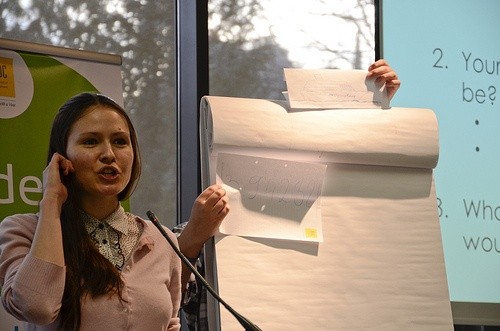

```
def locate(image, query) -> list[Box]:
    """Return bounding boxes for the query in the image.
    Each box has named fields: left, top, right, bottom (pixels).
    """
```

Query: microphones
left=145, top=208, right=264, bottom=331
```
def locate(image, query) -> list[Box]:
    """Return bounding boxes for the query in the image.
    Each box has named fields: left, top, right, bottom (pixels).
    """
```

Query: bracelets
left=181, top=250, right=201, bottom=263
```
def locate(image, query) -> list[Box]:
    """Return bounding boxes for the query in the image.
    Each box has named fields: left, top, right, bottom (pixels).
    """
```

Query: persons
left=172, top=59, right=401, bottom=331
left=0, top=93, right=181, bottom=331
